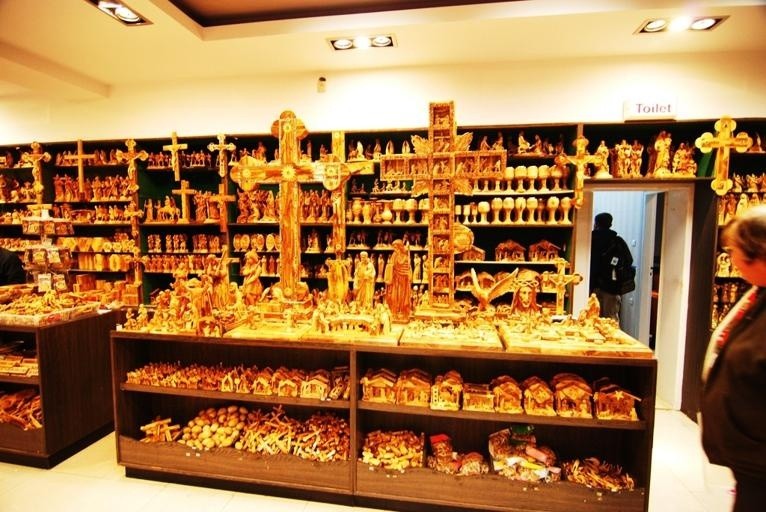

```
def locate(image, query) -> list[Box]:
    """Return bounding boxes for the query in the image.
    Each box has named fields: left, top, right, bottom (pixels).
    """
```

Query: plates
left=698, top=118, right=766, bottom=422
left=0, top=287, right=113, bottom=470
left=111, top=315, right=656, bottom=512
left=581, top=120, right=719, bottom=181
left=0, top=103, right=586, bottom=318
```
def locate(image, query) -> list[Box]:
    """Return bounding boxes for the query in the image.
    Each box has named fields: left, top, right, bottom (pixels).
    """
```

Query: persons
left=728, top=171, right=766, bottom=192
left=590, top=213, right=635, bottom=329
left=125, top=308, right=138, bottom=329
left=143, top=196, right=181, bottom=221
left=146, top=149, right=213, bottom=166
left=355, top=252, right=376, bottom=311
left=228, top=142, right=268, bottom=164
left=54, top=149, right=126, bottom=166
left=203, top=250, right=229, bottom=312
left=695, top=206, right=765, bottom=512
left=346, top=253, right=354, bottom=278
left=320, top=259, right=344, bottom=301
left=710, top=282, right=750, bottom=301
left=94, top=204, right=130, bottom=221
left=378, top=254, right=384, bottom=280
left=354, top=141, right=363, bottom=159
left=708, top=304, right=733, bottom=330
left=307, top=141, right=312, bottom=159
left=383, top=240, right=413, bottom=324
left=350, top=177, right=408, bottom=194
left=52, top=174, right=137, bottom=201
left=591, top=131, right=697, bottom=180
left=477, top=132, right=567, bottom=159
left=511, top=280, right=540, bottom=318
left=236, top=189, right=332, bottom=223
left=241, top=250, right=262, bottom=309
left=319, top=143, right=327, bottom=160
left=1, top=209, right=33, bottom=226
left=421, top=256, right=430, bottom=282
left=719, top=194, right=766, bottom=223
left=414, top=254, right=421, bottom=281
left=138, top=304, right=148, bottom=328
left=714, top=251, right=742, bottom=278
